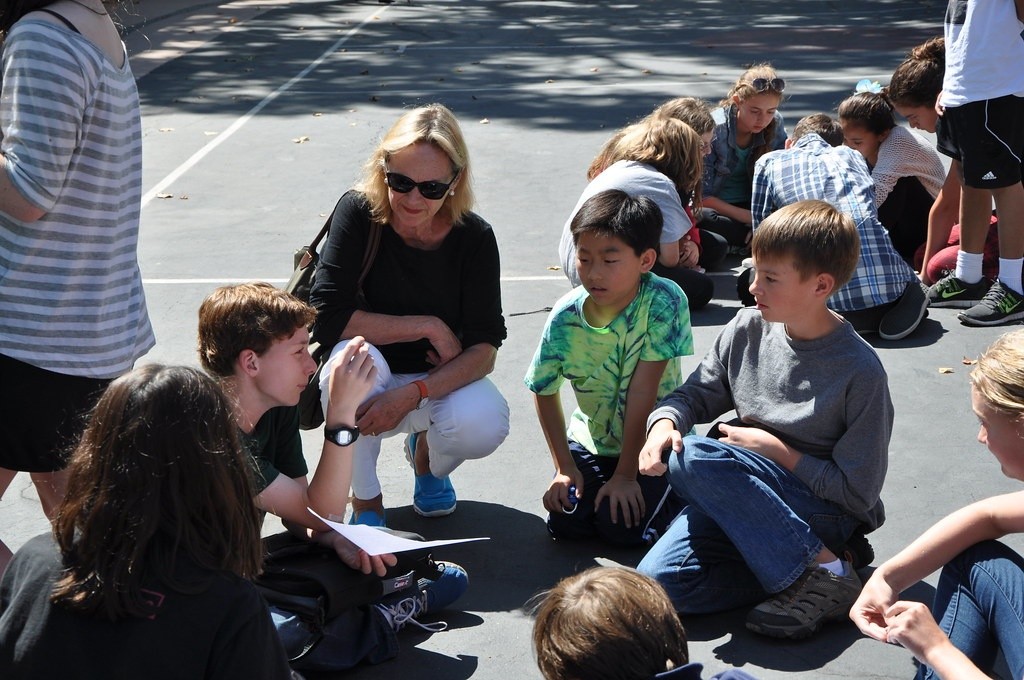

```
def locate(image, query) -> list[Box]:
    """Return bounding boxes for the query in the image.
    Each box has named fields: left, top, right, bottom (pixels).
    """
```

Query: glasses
left=384, top=168, right=459, bottom=200
left=700, top=135, right=717, bottom=150
left=736, top=78, right=785, bottom=93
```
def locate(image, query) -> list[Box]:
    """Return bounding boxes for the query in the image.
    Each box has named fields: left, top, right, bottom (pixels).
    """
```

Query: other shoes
left=839, top=307, right=889, bottom=334
left=880, top=282, right=931, bottom=339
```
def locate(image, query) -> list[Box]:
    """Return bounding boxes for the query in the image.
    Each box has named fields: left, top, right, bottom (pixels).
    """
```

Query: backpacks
left=242, top=522, right=438, bottom=675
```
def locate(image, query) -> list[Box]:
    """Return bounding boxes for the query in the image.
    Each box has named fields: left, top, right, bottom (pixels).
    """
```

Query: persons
left=194, top=282, right=469, bottom=671
left=524, top=190, right=695, bottom=549
left=312, top=105, right=511, bottom=528
left=0, top=363, right=291, bottom=680
left=558, top=98, right=716, bottom=311
left=0, top=0, right=157, bottom=580
left=849, top=327, right=1024, bottom=680
left=935, top=0, right=1024, bottom=325
left=639, top=200, right=894, bottom=639
left=890, top=38, right=1000, bottom=288
left=838, top=79, right=946, bottom=254
left=700, top=66, right=787, bottom=225
left=750, top=113, right=931, bottom=340
left=533, top=566, right=757, bottom=680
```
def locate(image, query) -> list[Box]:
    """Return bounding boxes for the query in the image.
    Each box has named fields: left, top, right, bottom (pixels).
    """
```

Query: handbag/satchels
left=284, top=191, right=383, bottom=430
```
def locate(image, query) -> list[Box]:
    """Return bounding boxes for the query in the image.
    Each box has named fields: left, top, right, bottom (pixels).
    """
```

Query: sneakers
left=373, top=557, right=471, bottom=634
left=959, top=275, right=1024, bottom=326
left=404, top=428, right=457, bottom=517
left=835, top=532, right=874, bottom=571
left=347, top=506, right=387, bottom=528
left=926, top=264, right=993, bottom=307
left=745, top=560, right=862, bottom=638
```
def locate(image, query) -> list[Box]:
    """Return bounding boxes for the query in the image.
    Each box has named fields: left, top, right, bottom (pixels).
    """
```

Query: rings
left=373, top=432, right=375, bottom=436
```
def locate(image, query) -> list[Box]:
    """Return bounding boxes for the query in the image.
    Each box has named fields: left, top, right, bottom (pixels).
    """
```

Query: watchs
left=324, top=424, right=359, bottom=446
left=413, top=380, right=429, bottom=410
